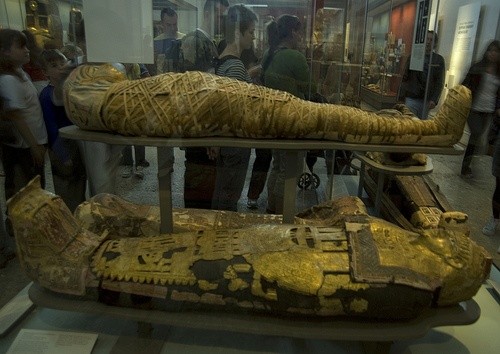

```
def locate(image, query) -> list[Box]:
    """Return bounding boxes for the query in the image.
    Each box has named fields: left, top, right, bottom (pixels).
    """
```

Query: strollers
left=297, top=148, right=358, bottom=190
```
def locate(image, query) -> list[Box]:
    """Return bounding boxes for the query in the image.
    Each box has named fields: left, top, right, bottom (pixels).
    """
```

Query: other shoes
left=264, top=208, right=282, bottom=215
left=121, top=165, right=133, bottom=178
left=459, top=173, right=473, bottom=179
left=482, top=216, right=500, bottom=236
left=134, top=166, right=144, bottom=177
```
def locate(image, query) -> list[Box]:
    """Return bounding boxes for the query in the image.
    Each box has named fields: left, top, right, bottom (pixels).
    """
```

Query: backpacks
left=156, top=31, right=212, bottom=75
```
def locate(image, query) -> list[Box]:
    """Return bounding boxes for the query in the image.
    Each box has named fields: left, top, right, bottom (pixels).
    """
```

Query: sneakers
left=247, top=197, right=258, bottom=208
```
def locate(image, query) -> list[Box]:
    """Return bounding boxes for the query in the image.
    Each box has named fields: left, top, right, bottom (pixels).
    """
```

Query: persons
left=402, top=29, right=446, bottom=121
left=61, top=64, right=472, bottom=147
left=484, top=90, right=500, bottom=236
left=6, top=174, right=493, bottom=323
left=455, top=39, right=500, bottom=175
left=0, top=0, right=311, bottom=212
